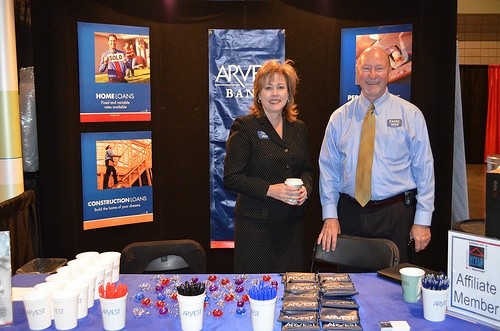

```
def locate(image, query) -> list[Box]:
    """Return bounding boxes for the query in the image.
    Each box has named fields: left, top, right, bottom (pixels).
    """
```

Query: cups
left=248, top=295, right=277, bottom=331
left=399, top=266, right=425, bottom=303
left=99, top=291, right=128, bottom=330
left=176, top=293, right=204, bottom=331
left=22, top=251, right=121, bottom=330
left=421, top=283, right=449, bottom=322
left=285, top=178, right=303, bottom=203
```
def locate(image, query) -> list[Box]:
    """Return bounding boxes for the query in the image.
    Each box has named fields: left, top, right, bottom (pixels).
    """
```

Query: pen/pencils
left=249, top=280, right=277, bottom=300
left=98, top=283, right=128, bottom=299
left=176, top=281, right=206, bottom=296
left=422, top=274, right=449, bottom=289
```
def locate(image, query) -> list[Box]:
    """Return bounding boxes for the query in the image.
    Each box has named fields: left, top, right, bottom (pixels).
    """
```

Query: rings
left=285, top=200, right=288, bottom=203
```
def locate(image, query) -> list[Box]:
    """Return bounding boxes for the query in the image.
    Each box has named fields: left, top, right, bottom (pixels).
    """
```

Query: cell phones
left=408, top=239, right=415, bottom=251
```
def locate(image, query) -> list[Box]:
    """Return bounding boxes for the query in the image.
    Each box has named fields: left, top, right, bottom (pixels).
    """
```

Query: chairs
left=309, top=235, right=400, bottom=273
left=120, top=240, right=207, bottom=274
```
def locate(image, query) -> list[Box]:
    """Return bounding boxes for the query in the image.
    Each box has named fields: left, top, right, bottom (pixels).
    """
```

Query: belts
left=340, top=188, right=416, bottom=210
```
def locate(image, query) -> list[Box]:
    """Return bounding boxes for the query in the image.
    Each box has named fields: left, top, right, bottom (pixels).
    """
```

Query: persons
left=103, top=145, right=121, bottom=190
left=124, top=44, right=145, bottom=70
left=99, top=34, right=131, bottom=83
left=317, top=46, right=435, bottom=273
left=225, top=59, right=314, bottom=274
left=123, top=42, right=135, bottom=78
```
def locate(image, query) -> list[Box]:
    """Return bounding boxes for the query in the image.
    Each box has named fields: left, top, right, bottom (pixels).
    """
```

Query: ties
left=354, top=103, right=377, bottom=208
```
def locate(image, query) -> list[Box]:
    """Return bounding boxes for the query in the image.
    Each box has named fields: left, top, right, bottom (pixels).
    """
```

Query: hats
left=105, top=143, right=110, bottom=149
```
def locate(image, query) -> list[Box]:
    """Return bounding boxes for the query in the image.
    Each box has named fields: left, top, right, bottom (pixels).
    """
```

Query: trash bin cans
left=15, top=257, right=67, bottom=275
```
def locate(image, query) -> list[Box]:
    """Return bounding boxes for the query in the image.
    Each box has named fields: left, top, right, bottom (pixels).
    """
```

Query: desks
left=0, top=271, right=497, bottom=331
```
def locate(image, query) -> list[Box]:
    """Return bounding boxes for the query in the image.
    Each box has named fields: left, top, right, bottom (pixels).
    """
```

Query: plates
left=377, top=263, right=441, bottom=281
